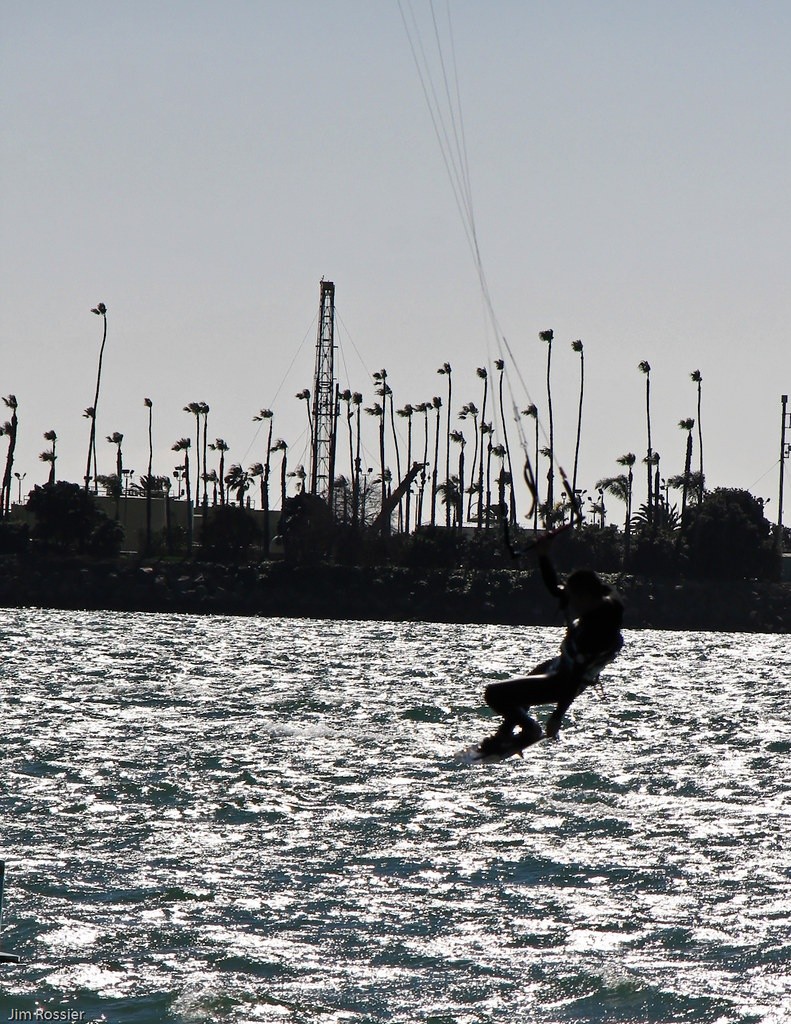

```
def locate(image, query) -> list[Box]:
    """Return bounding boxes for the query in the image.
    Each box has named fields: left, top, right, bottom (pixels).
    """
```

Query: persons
left=484, top=568, right=624, bottom=755
